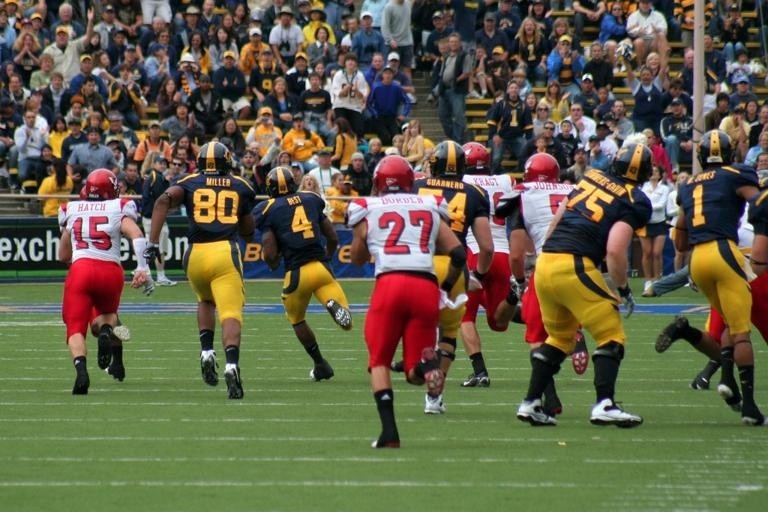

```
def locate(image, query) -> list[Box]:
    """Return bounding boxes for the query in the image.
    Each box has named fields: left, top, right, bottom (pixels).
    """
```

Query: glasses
left=545, top=126, right=554, bottom=131
left=539, top=108, right=547, bottom=111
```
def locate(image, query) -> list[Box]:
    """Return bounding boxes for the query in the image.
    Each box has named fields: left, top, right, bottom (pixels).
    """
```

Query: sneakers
left=655, top=317, right=688, bottom=354
left=73, top=376, right=90, bottom=395
left=461, top=371, right=490, bottom=388
left=407, top=92, right=415, bottom=101
left=516, top=396, right=562, bottom=425
left=390, top=347, right=446, bottom=414
left=98, top=326, right=131, bottom=381
left=589, top=398, right=642, bottom=428
left=154, top=278, right=177, bottom=286
left=572, top=330, right=588, bottom=374
left=689, top=375, right=768, bottom=426
left=371, top=433, right=399, bottom=449
left=310, top=360, right=334, bottom=381
left=201, top=350, right=219, bottom=386
left=427, top=94, right=434, bottom=101
left=326, top=300, right=352, bottom=328
left=224, top=363, right=243, bottom=399
left=642, top=279, right=660, bottom=297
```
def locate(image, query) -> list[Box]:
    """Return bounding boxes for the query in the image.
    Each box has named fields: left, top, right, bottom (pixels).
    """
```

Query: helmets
left=79, top=168, right=117, bottom=201
left=373, top=155, right=414, bottom=193
left=197, top=142, right=232, bottom=176
left=428, top=141, right=465, bottom=180
left=524, top=153, right=561, bottom=183
left=696, top=129, right=734, bottom=164
left=614, top=143, right=653, bottom=181
left=266, top=166, right=297, bottom=195
left=462, top=142, right=490, bottom=174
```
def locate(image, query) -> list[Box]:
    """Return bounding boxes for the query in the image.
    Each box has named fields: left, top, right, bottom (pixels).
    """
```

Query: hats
left=388, top=52, right=399, bottom=61
left=433, top=11, right=444, bottom=18
left=69, top=119, right=81, bottom=124
left=108, top=110, right=125, bottom=121
left=736, top=75, right=749, bottom=82
left=361, top=11, right=373, bottom=19
left=573, top=114, right=619, bottom=154
left=671, top=98, right=684, bottom=105
left=181, top=52, right=194, bottom=62
left=583, top=74, right=593, bottom=80
left=260, top=108, right=274, bottom=116
left=148, top=120, right=161, bottom=129
left=559, top=35, right=572, bottom=44
left=383, top=66, right=392, bottom=72
left=222, top=6, right=327, bottom=59
left=151, top=44, right=164, bottom=51
left=295, top=114, right=303, bottom=120
left=513, top=68, right=526, bottom=77
left=5, top=1, right=137, bottom=64
left=184, top=6, right=200, bottom=14
left=485, top=12, right=496, bottom=21
left=492, top=47, right=503, bottom=54
left=153, top=156, right=168, bottom=163
left=730, top=4, right=738, bottom=10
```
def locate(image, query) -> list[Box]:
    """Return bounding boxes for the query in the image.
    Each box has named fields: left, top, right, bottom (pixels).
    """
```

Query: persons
left=55, top=158, right=156, bottom=397
left=139, top=158, right=180, bottom=288
left=654, top=130, right=767, bottom=427
left=0, top=3, right=768, bottom=210
left=346, top=131, right=653, bottom=451
left=142, top=143, right=259, bottom=406
left=249, top=160, right=354, bottom=383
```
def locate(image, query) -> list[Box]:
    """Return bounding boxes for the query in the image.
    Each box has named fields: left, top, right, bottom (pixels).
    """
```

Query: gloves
left=510, top=276, right=530, bottom=299
left=616, top=284, right=634, bottom=319
